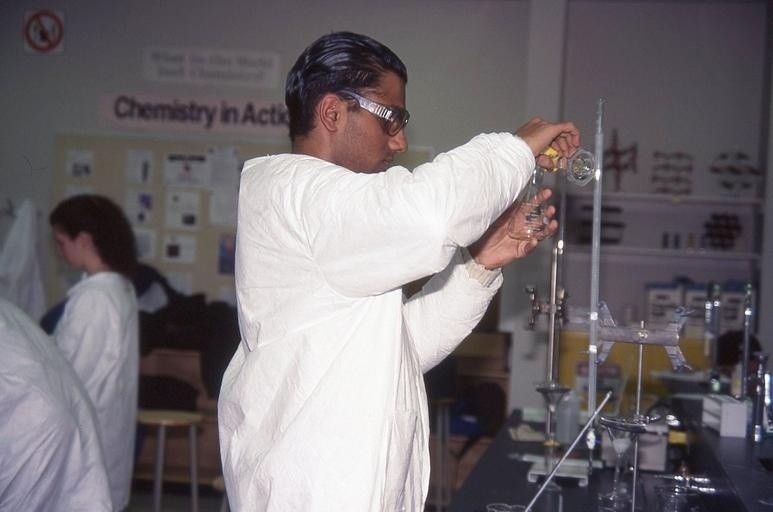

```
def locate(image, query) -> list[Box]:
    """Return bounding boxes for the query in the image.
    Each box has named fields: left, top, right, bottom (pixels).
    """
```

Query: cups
left=654, top=484, right=699, bottom=511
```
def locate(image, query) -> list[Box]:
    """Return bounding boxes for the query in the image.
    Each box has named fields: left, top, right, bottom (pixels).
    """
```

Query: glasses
left=336, top=89, right=410, bottom=136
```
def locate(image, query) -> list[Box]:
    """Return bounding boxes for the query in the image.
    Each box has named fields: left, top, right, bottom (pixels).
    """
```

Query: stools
left=132, top=398, right=204, bottom=512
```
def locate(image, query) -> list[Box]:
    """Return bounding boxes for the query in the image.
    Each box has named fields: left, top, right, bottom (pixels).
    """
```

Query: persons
left=0, top=288, right=115, bottom=512
left=49, top=192, right=142, bottom=511
left=717, top=329, right=764, bottom=395
left=215, top=27, right=582, bottom=512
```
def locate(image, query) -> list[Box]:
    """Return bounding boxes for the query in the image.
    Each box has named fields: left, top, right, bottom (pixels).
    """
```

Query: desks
left=445, top=408, right=768, bottom=510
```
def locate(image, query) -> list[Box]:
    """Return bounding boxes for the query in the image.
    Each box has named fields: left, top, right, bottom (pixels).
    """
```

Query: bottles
left=507, top=167, right=554, bottom=241
left=554, top=147, right=595, bottom=188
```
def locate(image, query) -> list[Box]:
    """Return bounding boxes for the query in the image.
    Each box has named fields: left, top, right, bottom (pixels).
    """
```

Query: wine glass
left=536, top=379, right=572, bottom=493
left=599, top=414, right=638, bottom=503
